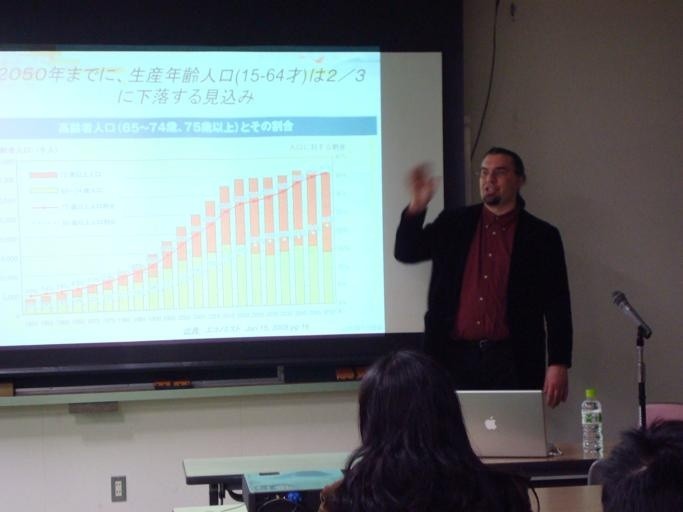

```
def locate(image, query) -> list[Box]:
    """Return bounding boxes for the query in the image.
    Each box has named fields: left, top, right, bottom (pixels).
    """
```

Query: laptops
left=454, top=389, right=551, bottom=459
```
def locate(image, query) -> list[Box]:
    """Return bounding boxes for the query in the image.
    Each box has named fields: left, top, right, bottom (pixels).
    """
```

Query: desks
left=170, top=442, right=619, bottom=511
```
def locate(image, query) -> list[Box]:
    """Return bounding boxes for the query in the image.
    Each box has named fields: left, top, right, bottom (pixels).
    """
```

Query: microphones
left=611, top=290, right=652, bottom=339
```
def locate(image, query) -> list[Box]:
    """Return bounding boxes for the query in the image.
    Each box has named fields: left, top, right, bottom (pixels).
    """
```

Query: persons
left=394, top=147, right=573, bottom=408
left=600, top=416, right=682, bottom=511
left=317, top=350, right=541, bottom=511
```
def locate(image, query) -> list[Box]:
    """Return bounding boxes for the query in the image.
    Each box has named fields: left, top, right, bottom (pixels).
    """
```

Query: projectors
left=240, top=466, right=345, bottom=512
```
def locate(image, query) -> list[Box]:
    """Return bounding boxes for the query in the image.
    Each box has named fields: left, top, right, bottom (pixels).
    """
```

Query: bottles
left=581, top=387, right=604, bottom=455
